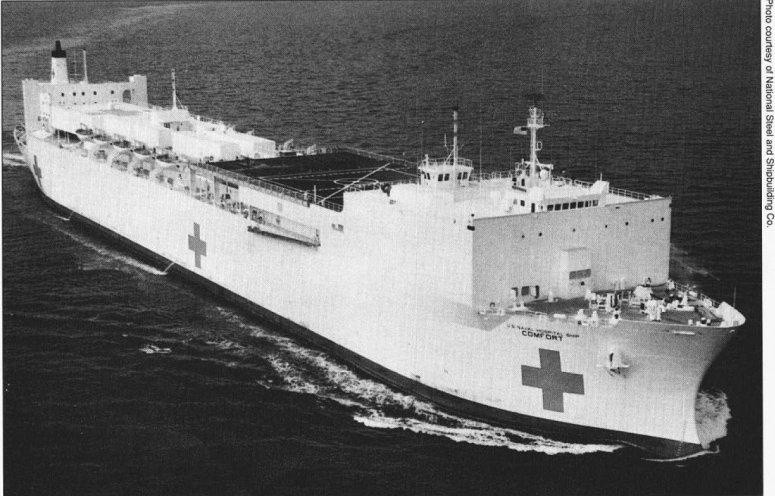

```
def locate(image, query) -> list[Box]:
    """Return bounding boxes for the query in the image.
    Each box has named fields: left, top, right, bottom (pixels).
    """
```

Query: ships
left=13, top=37, right=749, bottom=458
